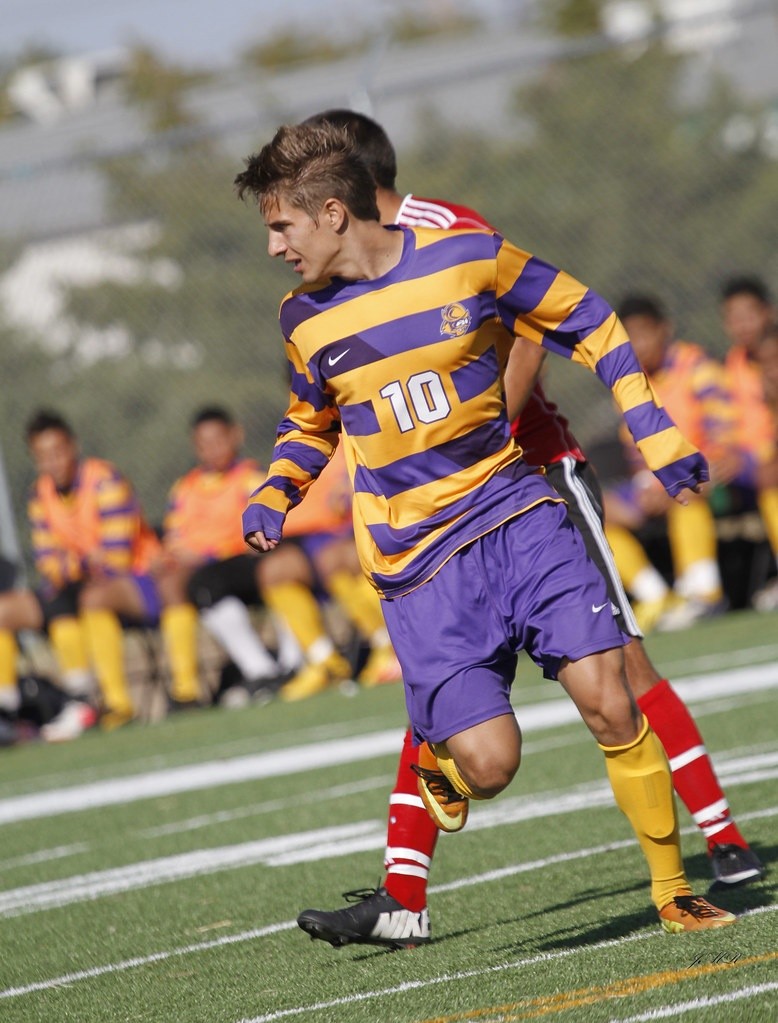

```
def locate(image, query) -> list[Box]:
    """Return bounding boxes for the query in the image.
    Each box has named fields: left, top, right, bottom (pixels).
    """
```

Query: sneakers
left=629, top=590, right=729, bottom=635
left=410, top=740, right=469, bottom=833
left=105, top=643, right=401, bottom=732
left=657, top=894, right=738, bottom=936
left=0, top=702, right=100, bottom=743
left=296, top=876, right=432, bottom=950
left=707, top=839, right=766, bottom=889
left=753, top=583, right=778, bottom=616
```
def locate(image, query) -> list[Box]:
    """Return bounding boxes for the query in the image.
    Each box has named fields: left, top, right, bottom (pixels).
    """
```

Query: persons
left=233, top=118, right=738, bottom=933
left=296, top=107, right=766, bottom=949
left=1, top=273, right=778, bottom=744
